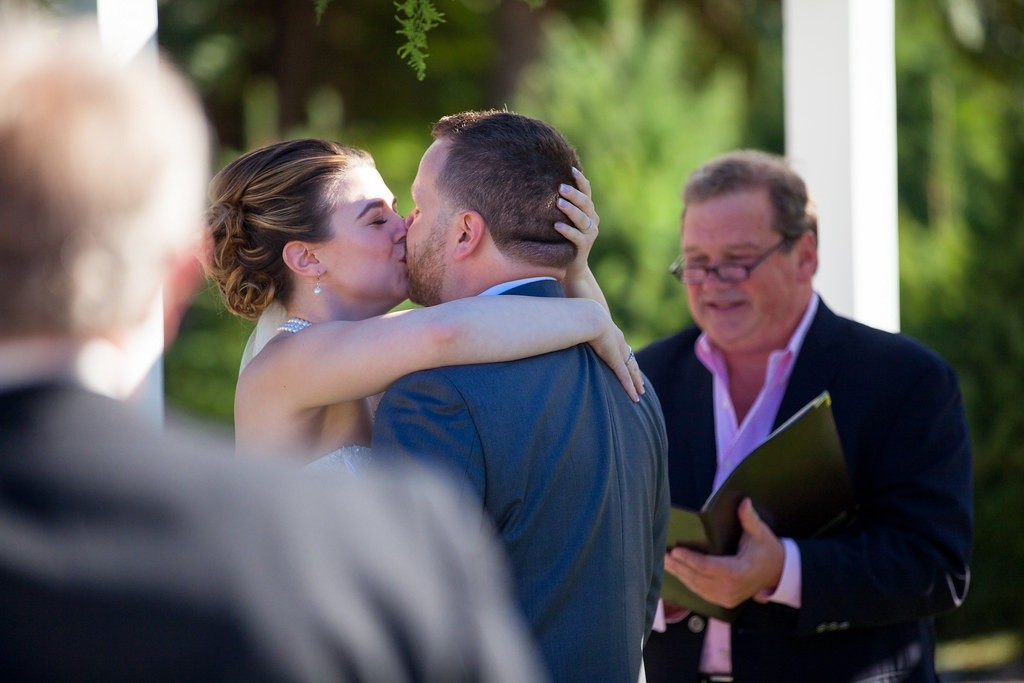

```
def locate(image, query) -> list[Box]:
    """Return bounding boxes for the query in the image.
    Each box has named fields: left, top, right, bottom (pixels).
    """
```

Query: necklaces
left=277, top=317, right=313, bottom=333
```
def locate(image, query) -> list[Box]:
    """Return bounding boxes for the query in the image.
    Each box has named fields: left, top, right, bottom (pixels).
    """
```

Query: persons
left=633, top=149, right=973, bottom=683
left=205, top=113, right=672, bottom=683
left=0, top=21, right=557, bottom=683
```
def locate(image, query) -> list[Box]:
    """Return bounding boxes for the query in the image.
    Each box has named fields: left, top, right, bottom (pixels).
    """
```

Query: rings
left=581, top=217, right=592, bottom=232
left=624, top=344, right=634, bottom=366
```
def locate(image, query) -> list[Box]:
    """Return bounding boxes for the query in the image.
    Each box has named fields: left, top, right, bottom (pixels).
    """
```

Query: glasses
left=669, top=237, right=788, bottom=285
